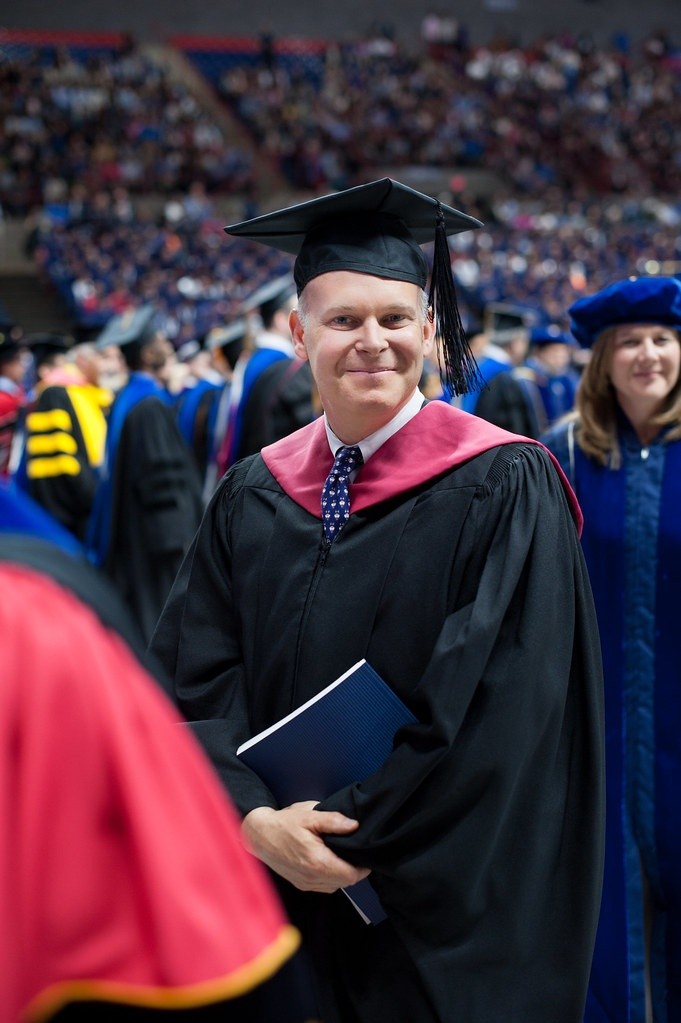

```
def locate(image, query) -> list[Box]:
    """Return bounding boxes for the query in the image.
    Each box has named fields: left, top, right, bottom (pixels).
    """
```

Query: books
left=236, top=658, right=417, bottom=926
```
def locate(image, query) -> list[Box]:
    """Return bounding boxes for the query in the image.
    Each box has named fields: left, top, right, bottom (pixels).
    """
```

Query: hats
left=565, top=274, right=681, bottom=348
left=0, top=258, right=565, bottom=393
left=223, top=177, right=484, bottom=395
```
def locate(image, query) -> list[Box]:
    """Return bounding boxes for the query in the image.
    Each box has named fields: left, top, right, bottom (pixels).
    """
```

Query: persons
left=0, top=13, right=681, bottom=563
left=0, top=530, right=304, bottom=1023
left=541, top=276, right=681, bottom=1023
left=147, top=177, right=608, bottom=1023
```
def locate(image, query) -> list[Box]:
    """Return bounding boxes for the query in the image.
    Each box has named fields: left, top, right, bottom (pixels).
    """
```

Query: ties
left=321, top=445, right=364, bottom=546
